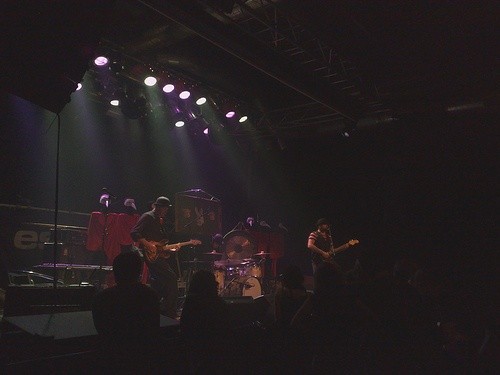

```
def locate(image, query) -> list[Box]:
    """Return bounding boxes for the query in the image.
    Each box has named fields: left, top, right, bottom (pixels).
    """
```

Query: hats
left=154, top=196, right=173, bottom=208
left=316, top=218, right=329, bottom=226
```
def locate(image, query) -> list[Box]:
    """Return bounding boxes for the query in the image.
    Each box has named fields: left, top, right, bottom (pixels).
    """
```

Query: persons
left=91, top=250, right=500, bottom=375
left=308, top=218, right=335, bottom=280
left=197, top=234, right=224, bottom=282
left=130, top=196, right=182, bottom=321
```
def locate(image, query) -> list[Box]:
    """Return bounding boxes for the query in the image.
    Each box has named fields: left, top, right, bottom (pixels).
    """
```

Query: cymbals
left=201, top=250, right=224, bottom=256
left=222, top=230, right=258, bottom=261
left=253, top=251, right=272, bottom=255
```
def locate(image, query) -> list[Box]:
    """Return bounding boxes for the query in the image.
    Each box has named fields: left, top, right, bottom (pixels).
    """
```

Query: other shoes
left=165, top=310, right=180, bottom=320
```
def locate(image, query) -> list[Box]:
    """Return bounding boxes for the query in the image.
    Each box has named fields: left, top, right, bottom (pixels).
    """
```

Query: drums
left=229, top=275, right=265, bottom=306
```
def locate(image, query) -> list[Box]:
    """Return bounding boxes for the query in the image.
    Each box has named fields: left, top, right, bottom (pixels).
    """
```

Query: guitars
left=312, top=238, right=360, bottom=270
left=142, top=239, right=202, bottom=265
